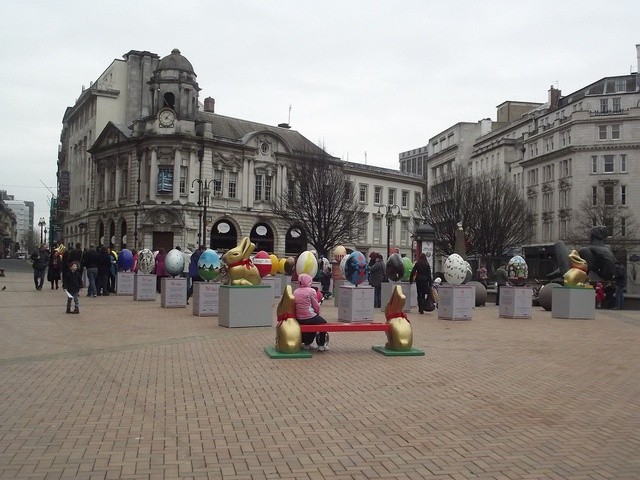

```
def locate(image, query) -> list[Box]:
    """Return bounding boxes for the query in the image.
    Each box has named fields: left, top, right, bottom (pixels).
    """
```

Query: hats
left=375, top=255, right=383, bottom=259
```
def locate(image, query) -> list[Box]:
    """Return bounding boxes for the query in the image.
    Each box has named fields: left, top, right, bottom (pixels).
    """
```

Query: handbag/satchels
left=424, top=294, right=435, bottom=311
left=382, top=275, right=389, bottom=282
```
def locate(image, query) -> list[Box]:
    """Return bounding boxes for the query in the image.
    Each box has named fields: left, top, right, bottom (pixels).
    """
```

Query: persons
left=595, top=282, right=606, bottom=307
left=368, top=255, right=387, bottom=308
left=293, top=273, right=329, bottom=351
left=156, top=248, right=169, bottom=293
left=63, top=262, right=84, bottom=315
left=320, top=267, right=331, bottom=291
left=368, top=251, right=378, bottom=266
left=69, top=243, right=83, bottom=261
left=603, top=281, right=616, bottom=308
left=30, top=244, right=51, bottom=290
left=175, top=246, right=181, bottom=251
left=85, top=244, right=117, bottom=298
left=182, top=247, right=194, bottom=304
left=187, top=245, right=206, bottom=305
left=410, top=253, right=433, bottom=314
left=132, top=248, right=139, bottom=273
left=612, top=262, right=626, bottom=309
left=48, top=251, right=62, bottom=290
left=495, top=263, right=514, bottom=305
left=63, top=247, right=73, bottom=261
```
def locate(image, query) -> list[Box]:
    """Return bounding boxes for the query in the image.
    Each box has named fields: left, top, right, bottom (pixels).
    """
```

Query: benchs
left=265, top=285, right=426, bottom=357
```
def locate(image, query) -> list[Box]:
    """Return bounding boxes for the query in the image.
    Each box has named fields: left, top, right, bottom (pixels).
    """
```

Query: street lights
left=376, top=205, right=402, bottom=259
left=190, top=178, right=223, bottom=249
left=38, top=217, right=46, bottom=244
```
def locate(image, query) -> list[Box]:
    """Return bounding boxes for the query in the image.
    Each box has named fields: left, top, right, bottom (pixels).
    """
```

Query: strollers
left=316, top=291, right=333, bottom=346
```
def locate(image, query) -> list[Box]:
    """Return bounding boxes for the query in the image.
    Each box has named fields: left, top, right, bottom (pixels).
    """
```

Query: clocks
left=158, top=108, right=176, bottom=128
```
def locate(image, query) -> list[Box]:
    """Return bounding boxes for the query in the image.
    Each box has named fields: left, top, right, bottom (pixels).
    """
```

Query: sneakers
left=66, top=310, right=72, bottom=313
left=304, top=343, right=317, bottom=350
left=318, top=343, right=328, bottom=351
left=72, top=310, right=79, bottom=314
